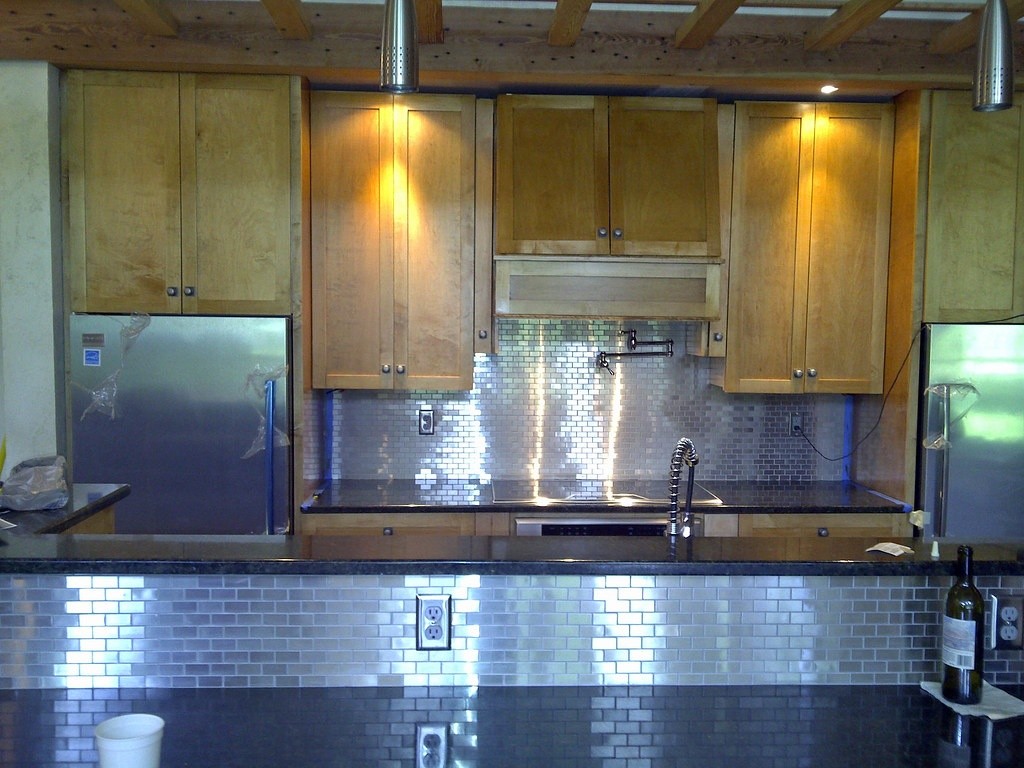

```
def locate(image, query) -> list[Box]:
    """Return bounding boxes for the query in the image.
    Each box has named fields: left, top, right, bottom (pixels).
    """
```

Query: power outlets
left=419, top=409, right=434, bottom=435
left=415, top=723, right=449, bottom=767
left=415, top=594, right=452, bottom=651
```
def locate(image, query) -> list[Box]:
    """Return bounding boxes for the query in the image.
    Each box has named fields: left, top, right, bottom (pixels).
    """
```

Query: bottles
left=943, top=546, right=986, bottom=704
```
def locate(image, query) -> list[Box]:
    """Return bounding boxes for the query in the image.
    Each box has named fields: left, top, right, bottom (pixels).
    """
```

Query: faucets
left=666, top=438, right=700, bottom=549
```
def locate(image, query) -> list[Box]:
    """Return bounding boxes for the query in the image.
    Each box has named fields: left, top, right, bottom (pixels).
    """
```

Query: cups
left=95, top=714, right=165, bottom=768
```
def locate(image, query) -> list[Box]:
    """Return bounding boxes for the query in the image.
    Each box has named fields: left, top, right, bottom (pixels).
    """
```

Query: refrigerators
left=920, top=321, right=1024, bottom=542
left=71, top=314, right=294, bottom=537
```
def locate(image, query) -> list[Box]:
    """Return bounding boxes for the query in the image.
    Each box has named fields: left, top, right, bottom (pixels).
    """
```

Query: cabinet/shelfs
left=923, top=89, right=1024, bottom=325
left=67, top=67, right=292, bottom=316
left=493, top=90, right=722, bottom=259
left=309, top=89, right=473, bottom=391
left=711, top=100, right=895, bottom=395
left=301, top=514, right=915, bottom=538
